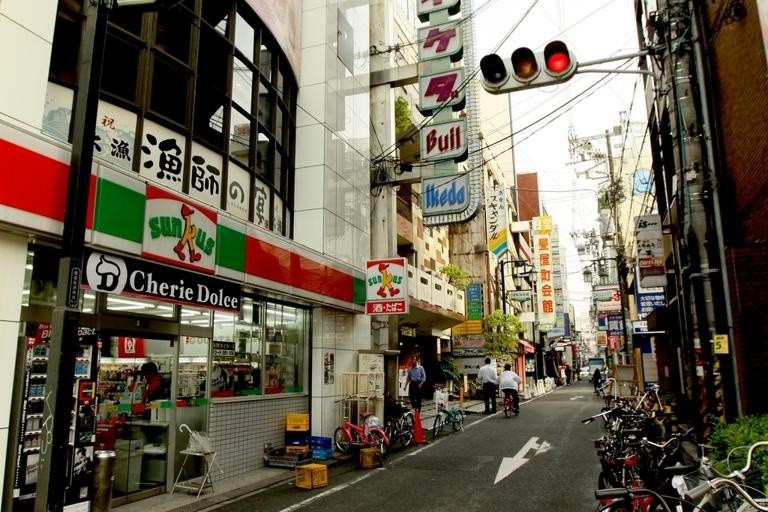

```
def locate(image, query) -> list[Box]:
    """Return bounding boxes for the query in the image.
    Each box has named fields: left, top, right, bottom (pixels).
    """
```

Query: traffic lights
left=476, top=38, right=579, bottom=94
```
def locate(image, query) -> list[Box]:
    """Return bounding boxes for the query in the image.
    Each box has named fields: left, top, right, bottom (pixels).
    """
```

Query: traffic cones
left=408, top=407, right=424, bottom=446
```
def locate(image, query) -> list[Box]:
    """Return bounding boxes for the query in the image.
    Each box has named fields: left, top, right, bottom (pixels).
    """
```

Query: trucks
left=588, top=356, right=606, bottom=381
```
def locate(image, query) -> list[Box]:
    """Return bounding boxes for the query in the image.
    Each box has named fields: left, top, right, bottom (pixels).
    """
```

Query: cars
left=579, top=367, right=589, bottom=381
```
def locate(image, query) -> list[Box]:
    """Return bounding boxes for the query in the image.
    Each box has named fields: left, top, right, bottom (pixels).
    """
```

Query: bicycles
left=582, top=375, right=768, bottom=511
left=376, top=392, right=416, bottom=455
left=501, top=382, right=520, bottom=418
left=332, top=391, right=391, bottom=457
left=427, top=384, right=468, bottom=437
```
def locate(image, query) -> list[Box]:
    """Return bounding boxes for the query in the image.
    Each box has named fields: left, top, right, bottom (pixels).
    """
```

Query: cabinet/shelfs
left=169, top=449, right=216, bottom=499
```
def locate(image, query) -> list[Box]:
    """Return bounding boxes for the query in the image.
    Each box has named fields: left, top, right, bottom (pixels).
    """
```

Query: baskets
left=387, top=402, right=406, bottom=419
left=311, top=436, right=333, bottom=460
left=433, top=392, right=449, bottom=404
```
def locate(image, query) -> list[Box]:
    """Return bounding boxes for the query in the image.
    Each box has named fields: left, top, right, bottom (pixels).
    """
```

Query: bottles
left=150, top=399, right=170, bottom=421
left=99, top=365, right=128, bottom=379
left=220, top=367, right=249, bottom=389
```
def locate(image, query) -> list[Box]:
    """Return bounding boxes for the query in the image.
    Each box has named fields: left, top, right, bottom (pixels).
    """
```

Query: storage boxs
left=284, top=411, right=383, bottom=491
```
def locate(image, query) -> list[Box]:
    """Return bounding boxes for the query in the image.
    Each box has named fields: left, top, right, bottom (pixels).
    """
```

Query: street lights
left=29, top=0, right=165, bottom=510
left=578, top=254, right=630, bottom=367
left=499, top=258, right=526, bottom=355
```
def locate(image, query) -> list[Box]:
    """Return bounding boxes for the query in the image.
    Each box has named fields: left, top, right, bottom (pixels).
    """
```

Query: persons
left=497, top=364, right=523, bottom=416
left=592, top=368, right=602, bottom=393
left=324, top=357, right=334, bottom=383
left=330, top=357, right=334, bottom=384
left=140, top=363, right=166, bottom=404
left=403, top=355, right=426, bottom=414
left=477, top=357, right=497, bottom=415
left=558, top=363, right=571, bottom=386
